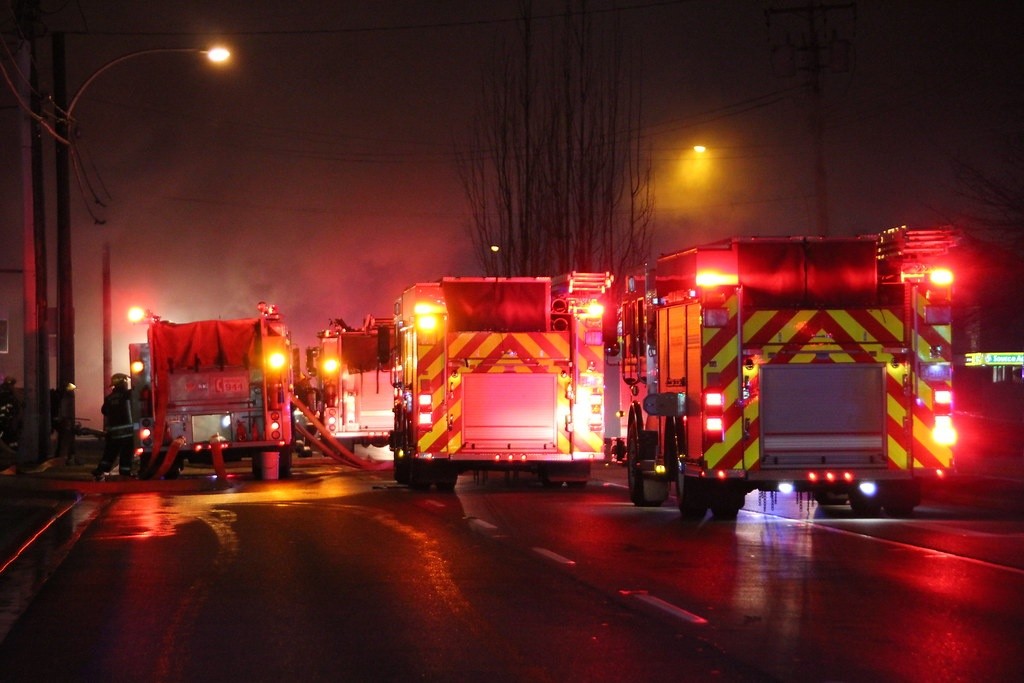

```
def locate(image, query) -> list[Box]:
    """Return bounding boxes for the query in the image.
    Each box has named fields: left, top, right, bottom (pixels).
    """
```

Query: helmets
left=4, top=376, right=16, bottom=384
left=111, top=373, right=127, bottom=386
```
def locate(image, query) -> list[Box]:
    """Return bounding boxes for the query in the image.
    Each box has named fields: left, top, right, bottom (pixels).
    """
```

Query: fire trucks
left=376, top=271, right=614, bottom=495
left=128, top=302, right=295, bottom=480
left=305, top=313, right=397, bottom=456
left=603, top=225, right=959, bottom=524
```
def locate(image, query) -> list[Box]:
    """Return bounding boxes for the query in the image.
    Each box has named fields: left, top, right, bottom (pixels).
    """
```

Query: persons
left=0, top=377, right=20, bottom=455
left=92, top=373, right=137, bottom=480
left=292, top=371, right=323, bottom=458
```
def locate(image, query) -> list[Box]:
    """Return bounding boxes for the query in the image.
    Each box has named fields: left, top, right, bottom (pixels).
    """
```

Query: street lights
left=55, top=46, right=231, bottom=455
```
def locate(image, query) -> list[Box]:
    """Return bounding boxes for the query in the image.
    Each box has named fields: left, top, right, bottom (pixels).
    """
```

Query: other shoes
left=118, top=470, right=131, bottom=476
left=90, top=469, right=104, bottom=477
